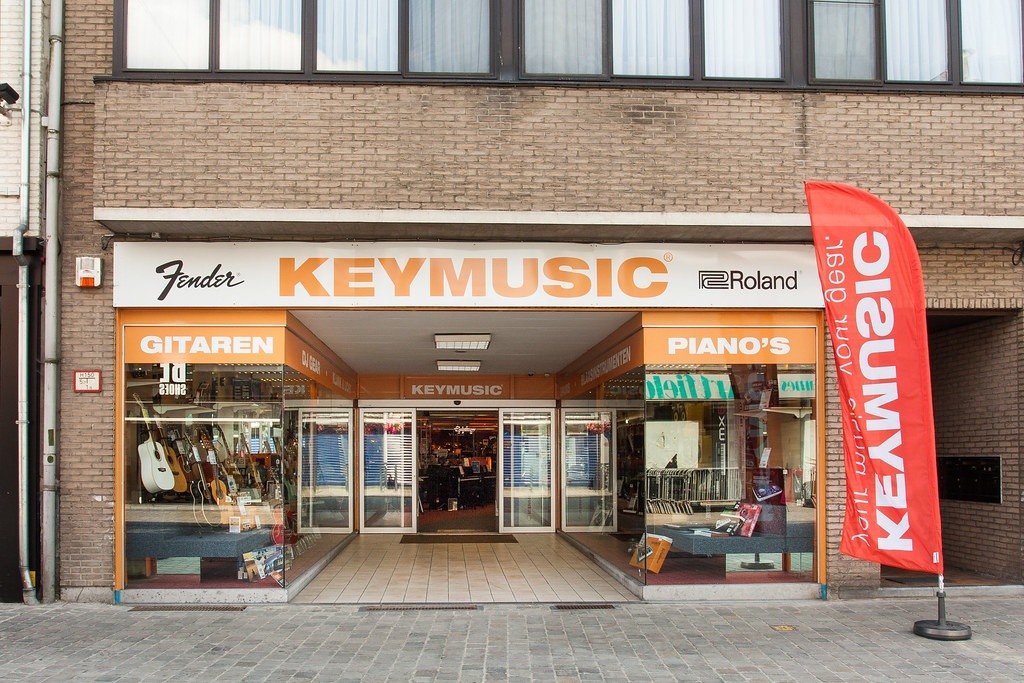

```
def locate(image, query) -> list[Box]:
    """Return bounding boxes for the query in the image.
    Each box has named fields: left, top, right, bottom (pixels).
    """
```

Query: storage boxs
left=628, top=532, right=673, bottom=574
left=240, top=544, right=287, bottom=582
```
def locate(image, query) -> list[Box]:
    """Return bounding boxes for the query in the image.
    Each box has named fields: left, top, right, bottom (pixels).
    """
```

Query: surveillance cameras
left=529, top=372, right=535, bottom=377
left=454, top=400, right=461, bottom=405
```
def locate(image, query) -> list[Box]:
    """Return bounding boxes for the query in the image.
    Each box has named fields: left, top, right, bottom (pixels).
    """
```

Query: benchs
left=125, top=524, right=270, bottom=577
left=646, top=523, right=815, bottom=572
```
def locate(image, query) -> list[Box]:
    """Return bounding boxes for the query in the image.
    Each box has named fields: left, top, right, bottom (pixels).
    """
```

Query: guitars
left=133, top=397, right=299, bottom=544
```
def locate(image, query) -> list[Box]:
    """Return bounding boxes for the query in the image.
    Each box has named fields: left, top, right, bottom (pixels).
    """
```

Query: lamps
left=0, top=82, right=19, bottom=114
left=434, top=334, right=492, bottom=350
left=436, top=360, right=481, bottom=372
